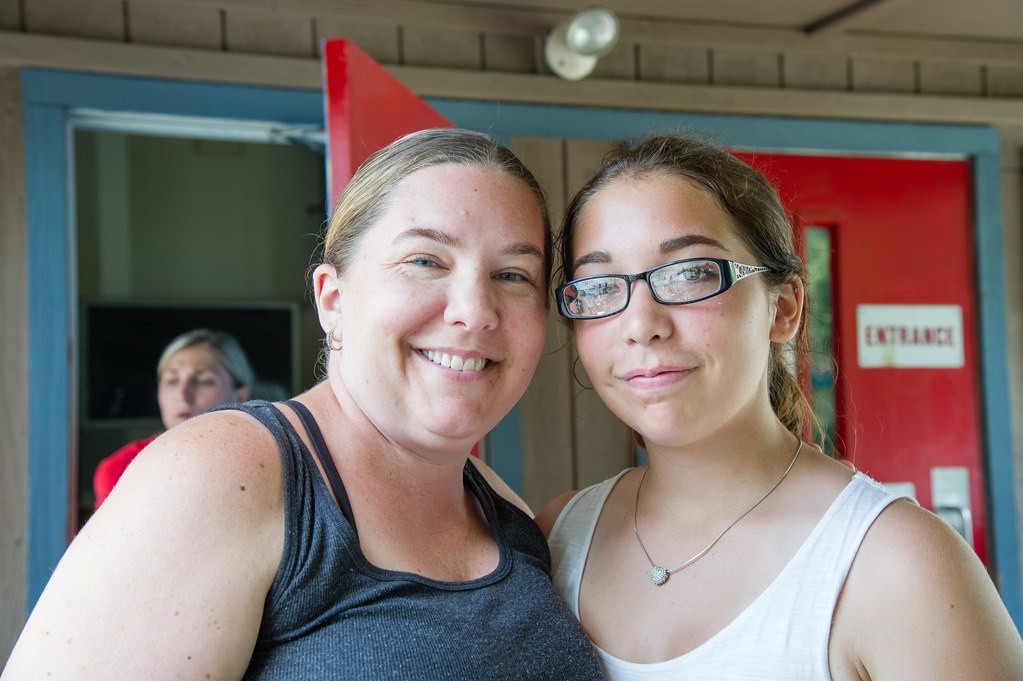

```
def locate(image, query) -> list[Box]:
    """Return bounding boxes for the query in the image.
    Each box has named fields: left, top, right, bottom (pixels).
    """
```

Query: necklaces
left=631, top=431, right=803, bottom=585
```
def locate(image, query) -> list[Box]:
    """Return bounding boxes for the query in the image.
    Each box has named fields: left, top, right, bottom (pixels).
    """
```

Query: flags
left=325, top=35, right=454, bottom=218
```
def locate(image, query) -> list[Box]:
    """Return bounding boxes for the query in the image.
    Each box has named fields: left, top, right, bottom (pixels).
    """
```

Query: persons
left=91, top=327, right=253, bottom=514
left=532, top=135, right=1023, bottom=681
left=0, top=127, right=610, bottom=681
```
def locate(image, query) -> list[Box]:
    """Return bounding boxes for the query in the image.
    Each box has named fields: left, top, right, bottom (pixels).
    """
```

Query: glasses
left=554, top=256, right=776, bottom=321
